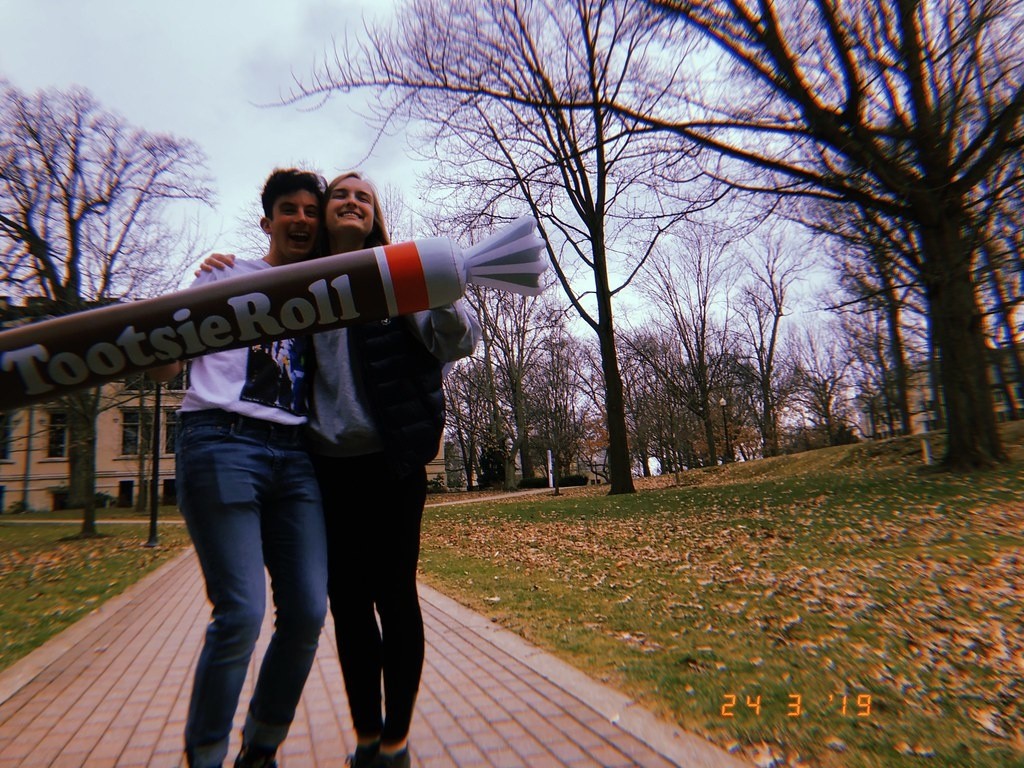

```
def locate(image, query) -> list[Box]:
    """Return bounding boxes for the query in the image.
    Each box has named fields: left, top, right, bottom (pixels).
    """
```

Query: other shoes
left=350, top=741, right=380, bottom=768
left=234, top=749, right=277, bottom=768
left=374, top=750, right=409, bottom=768
left=185, top=749, right=221, bottom=768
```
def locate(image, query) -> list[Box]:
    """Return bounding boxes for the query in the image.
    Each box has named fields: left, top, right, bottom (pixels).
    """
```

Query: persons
left=194, top=171, right=484, bottom=768
left=146, top=166, right=331, bottom=768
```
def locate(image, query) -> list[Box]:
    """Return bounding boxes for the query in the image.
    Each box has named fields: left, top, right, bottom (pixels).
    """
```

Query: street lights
left=719, top=398, right=732, bottom=463
